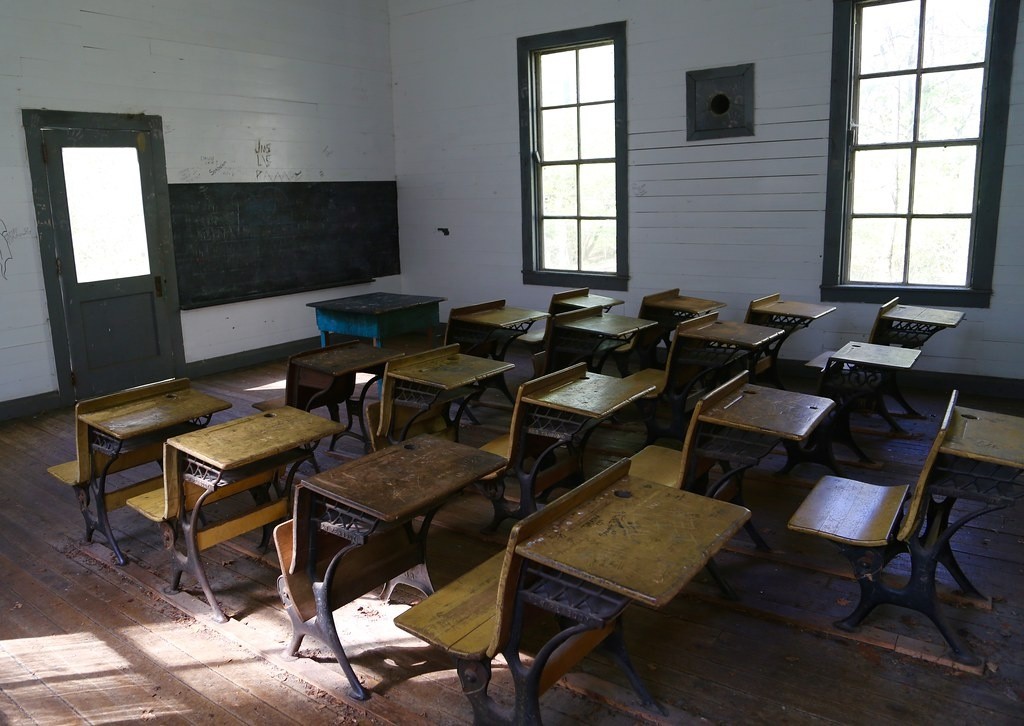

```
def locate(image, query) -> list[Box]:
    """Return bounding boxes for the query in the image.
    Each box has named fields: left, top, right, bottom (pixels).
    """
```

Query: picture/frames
left=685, top=62, right=755, bottom=142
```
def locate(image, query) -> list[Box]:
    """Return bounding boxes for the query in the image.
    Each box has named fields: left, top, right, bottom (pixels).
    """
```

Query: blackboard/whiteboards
left=166, top=180, right=402, bottom=311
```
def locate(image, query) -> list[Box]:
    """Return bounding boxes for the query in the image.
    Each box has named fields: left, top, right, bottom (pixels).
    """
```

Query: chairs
left=785, top=388, right=989, bottom=676
left=394, top=456, right=668, bottom=726
left=709, top=295, right=787, bottom=388
left=770, top=357, right=877, bottom=478
left=471, top=361, right=587, bottom=537
left=366, top=343, right=465, bottom=517
left=443, top=298, right=515, bottom=426
left=124, top=442, right=314, bottom=624
left=46, top=378, right=210, bottom=567
left=532, top=306, right=618, bottom=428
left=272, top=485, right=436, bottom=703
left=511, top=287, right=590, bottom=351
left=531, top=305, right=620, bottom=449
left=629, top=370, right=773, bottom=602
left=251, top=339, right=372, bottom=476
left=805, top=296, right=922, bottom=434
left=599, top=287, right=680, bottom=379
left=622, top=311, right=719, bottom=452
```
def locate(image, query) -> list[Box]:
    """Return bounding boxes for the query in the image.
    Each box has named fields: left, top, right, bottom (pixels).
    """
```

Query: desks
left=166, top=404, right=347, bottom=493
left=675, top=320, right=785, bottom=368
left=555, top=313, right=659, bottom=354
left=692, top=383, right=836, bottom=467
left=874, top=304, right=967, bottom=347
left=644, top=294, right=727, bottom=331
left=300, top=432, right=509, bottom=544
left=77, top=382, right=233, bottom=456
left=292, top=342, right=406, bottom=389
left=928, top=406, right=1024, bottom=507
left=515, top=475, right=751, bottom=631
left=552, top=293, right=625, bottom=318
left=387, top=353, right=516, bottom=412
left=830, top=340, right=922, bottom=397
left=306, top=292, right=448, bottom=400
left=520, top=371, right=656, bottom=445
left=748, top=300, right=837, bottom=334
left=449, top=306, right=552, bottom=346
